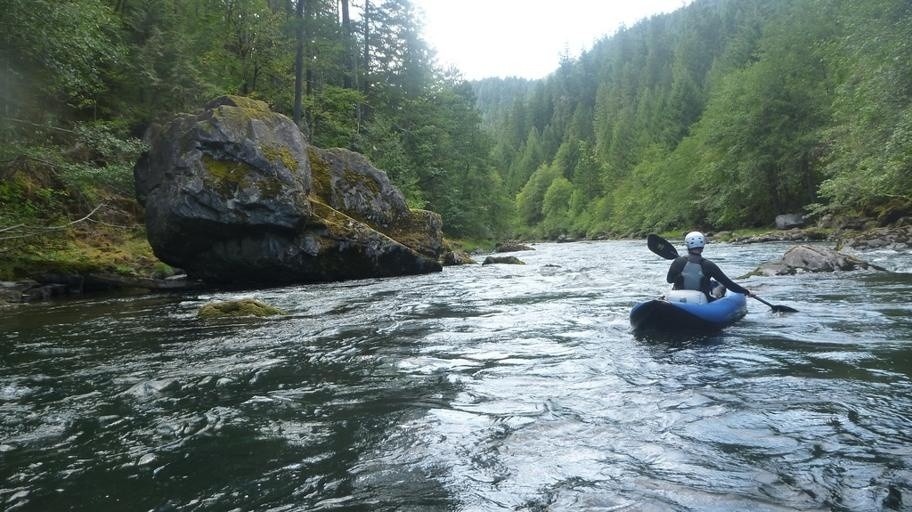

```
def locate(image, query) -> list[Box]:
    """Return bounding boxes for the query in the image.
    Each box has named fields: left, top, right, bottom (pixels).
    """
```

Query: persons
left=666, top=231, right=754, bottom=301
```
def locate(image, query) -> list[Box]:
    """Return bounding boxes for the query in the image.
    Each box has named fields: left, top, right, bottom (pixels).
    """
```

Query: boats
left=628, top=291, right=749, bottom=335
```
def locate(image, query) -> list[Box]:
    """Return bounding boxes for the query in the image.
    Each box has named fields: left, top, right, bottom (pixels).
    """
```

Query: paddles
left=647, top=235, right=799, bottom=314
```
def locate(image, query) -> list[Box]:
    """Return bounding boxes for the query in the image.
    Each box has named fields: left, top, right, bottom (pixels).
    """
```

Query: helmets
left=684, top=231, right=705, bottom=249
